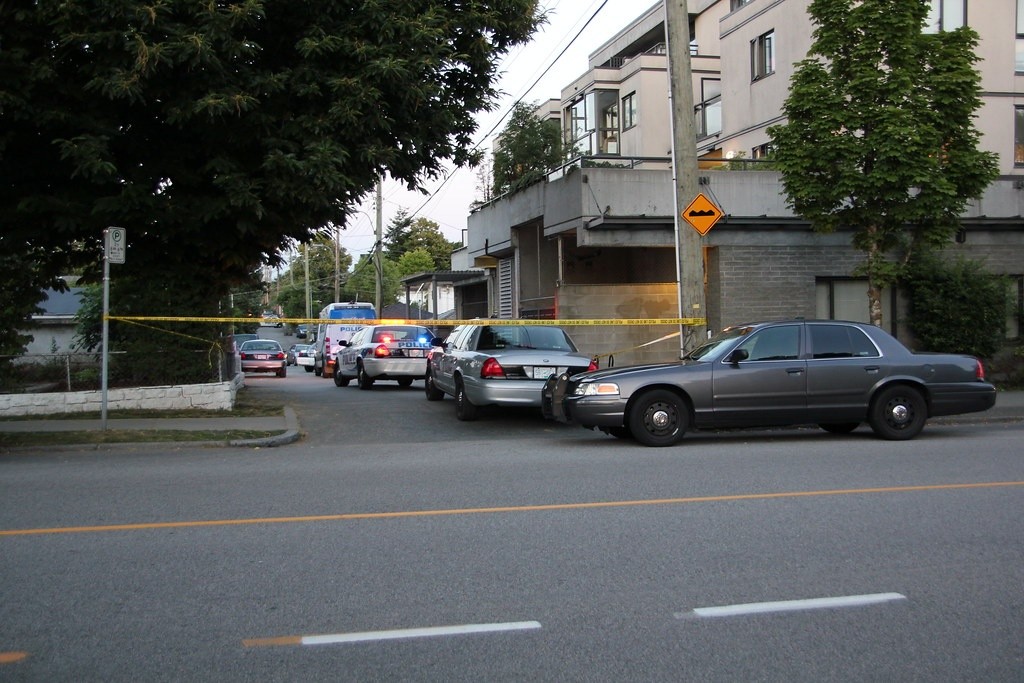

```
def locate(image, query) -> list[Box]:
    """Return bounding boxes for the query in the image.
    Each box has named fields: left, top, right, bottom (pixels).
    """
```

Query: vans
left=313, top=301, right=379, bottom=377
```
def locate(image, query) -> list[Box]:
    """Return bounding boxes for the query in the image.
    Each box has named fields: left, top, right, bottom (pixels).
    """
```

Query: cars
left=333, top=322, right=441, bottom=391
left=286, top=343, right=312, bottom=367
left=259, top=314, right=283, bottom=329
left=234, top=332, right=260, bottom=349
left=237, top=338, right=287, bottom=378
left=540, top=317, right=997, bottom=448
left=424, top=319, right=599, bottom=422
left=296, top=322, right=316, bottom=338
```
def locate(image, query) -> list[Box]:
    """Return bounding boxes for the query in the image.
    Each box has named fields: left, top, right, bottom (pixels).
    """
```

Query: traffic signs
left=107, top=226, right=125, bottom=264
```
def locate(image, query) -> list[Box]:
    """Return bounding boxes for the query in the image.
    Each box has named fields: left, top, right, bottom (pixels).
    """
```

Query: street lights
left=348, top=208, right=383, bottom=320
left=312, top=243, right=340, bottom=304
